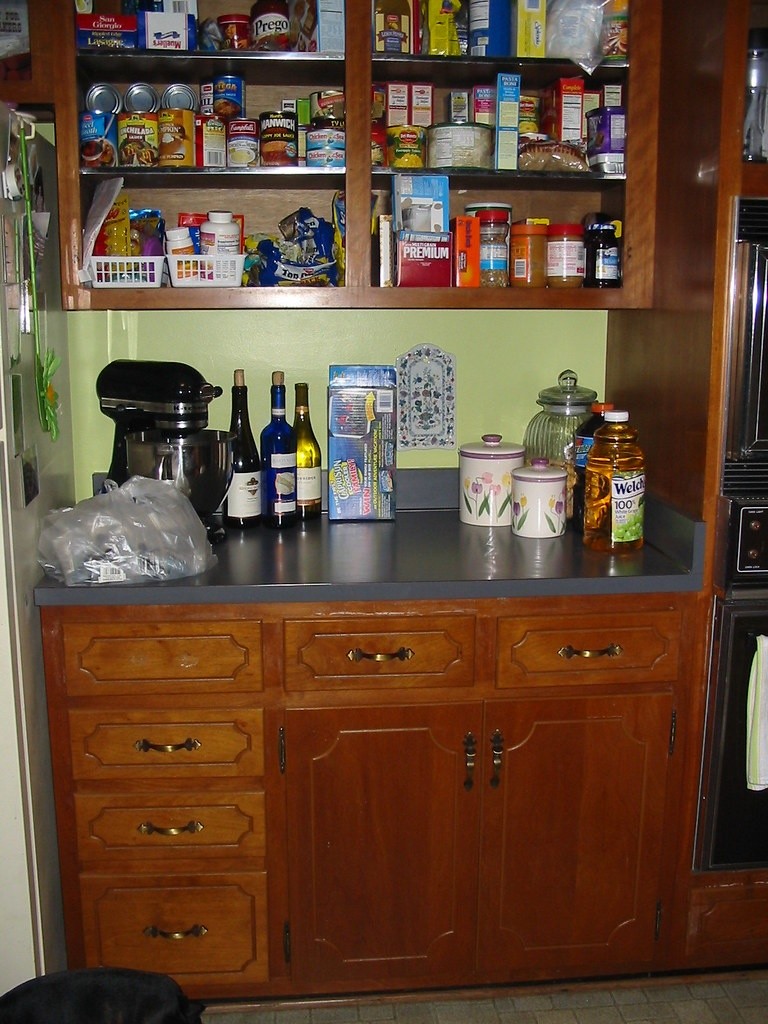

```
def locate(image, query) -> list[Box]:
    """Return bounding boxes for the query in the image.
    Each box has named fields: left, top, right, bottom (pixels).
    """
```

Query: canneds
left=585, top=223, right=622, bottom=287
left=79, top=75, right=306, bottom=167
left=464, top=203, right=512, bottom=272
left=426, top=122, right=495, bottom=169
left=546, top=223, right=585, bottom=286
left=305, top=89, right=345, bottom=166
left=386, top=124, right=428, bottom=168
left=217, top=0, right=291, bottom=51
left=474, top=209, right=510, bottom=287
left=510, top=224, right=550, bottom=287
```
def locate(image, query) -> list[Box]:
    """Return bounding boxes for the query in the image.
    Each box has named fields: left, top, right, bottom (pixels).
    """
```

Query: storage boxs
left=467, top=85, right=496, bottom=126
left=586, top=106, right=626, bottom=174
left=378, top=81, right=410, bottom=128
left=288, top=0, right=345, bottom=53
left=89, top=255, right=166, bottom=288
left=410, top=81, right=435, bottom=125
left=326, top=365, right=396, bottom=522
left=400, top=230, right=453, bottom=287
left=541, top=76, right=583, bottom=142
left=450, top=217, right=479, bottom=288
left=513, top=0, right=546, bottom=58
left=496, top=73, right=520, bottom=170
left=165, top=252, right=250, bottom=286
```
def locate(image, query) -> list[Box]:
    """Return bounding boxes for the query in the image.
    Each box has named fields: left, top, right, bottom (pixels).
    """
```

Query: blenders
left=92, top=360, right=237, bottom=536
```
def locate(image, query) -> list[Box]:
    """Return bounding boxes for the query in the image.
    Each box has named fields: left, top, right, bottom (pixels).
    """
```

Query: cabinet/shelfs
left=1, top=0, right=767, bottom=1001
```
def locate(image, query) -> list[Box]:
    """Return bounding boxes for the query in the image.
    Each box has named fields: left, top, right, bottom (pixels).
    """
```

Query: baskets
left=166, top=255, right=248, bottom=287
left=89, top=256, right=164, bottom=289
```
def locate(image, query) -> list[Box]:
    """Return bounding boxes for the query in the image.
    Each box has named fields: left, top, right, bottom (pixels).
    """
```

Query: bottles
left=574, top=403, right=613, bottom=532
left=742, top=49, right=768, bottom=163
left=476, top=210, right=509, bottom=288
left=602, top=0, right=628, bottom=65
left=586, top=224, right=621, bottom=288
left=585, top=410, right=646, bottom=552
left=523, top=369, right=599, bottom=518
left=547, top=223, right=585, bottom=286
left=293, top=384, right=320, bottom=521
left=222, top=370, right=262, bottom=530
left=259, top=369, right=297, bottom=529
left=200, top=210, right=240, bottom=281
left=165, top=228, right=197, bottom=281
left=511, top=225, right=546, bottom=286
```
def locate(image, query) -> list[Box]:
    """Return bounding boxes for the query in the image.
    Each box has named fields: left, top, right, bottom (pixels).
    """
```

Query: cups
left=458, top=434, right=525, bottom=527
left=512, top=459, right=569, bottom=539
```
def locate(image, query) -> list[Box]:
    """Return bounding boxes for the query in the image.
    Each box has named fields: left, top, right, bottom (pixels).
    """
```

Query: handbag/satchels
left=35, top=474, right=218, bottom=587
